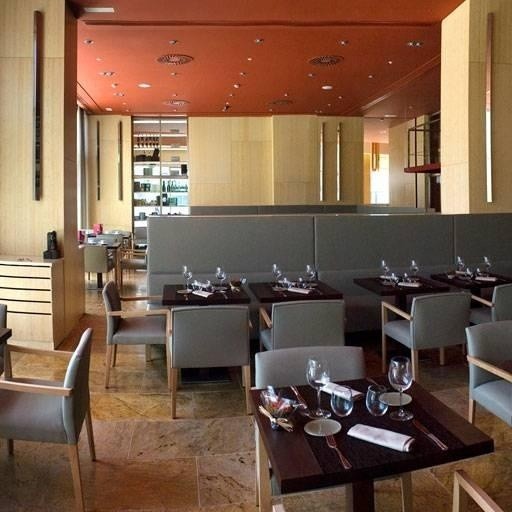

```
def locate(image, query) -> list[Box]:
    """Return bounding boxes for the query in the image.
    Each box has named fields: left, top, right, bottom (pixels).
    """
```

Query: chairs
left=0, top=303, right=11, bottom=377
left=80, top=229, right=133, bottom=289
left=462, top=285, right=512, bottom=355
left=256, top=299, right=349, bottom=352
left=0, top=328, right=100, bottom=511
left=101, top=281, right=173, bottom=390
left=465, top=320, right=512, bottom=430
left=447, top=466, right=511, bottom=511
left=381, top=292, right=471, bottom=382
left=254, top=347, right=415, bottom=512
left=167, top=304, right=251, bottom=417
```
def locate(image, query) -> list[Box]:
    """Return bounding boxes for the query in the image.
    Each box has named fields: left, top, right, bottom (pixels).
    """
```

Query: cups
left=332, top=385, right=353, bottom=415
left=87, top=229, right=121, bottom=246
left=366, top=383, right=387, bottom=416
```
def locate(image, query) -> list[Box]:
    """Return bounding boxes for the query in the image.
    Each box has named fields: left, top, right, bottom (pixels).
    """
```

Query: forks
left=324, top=431, right=353, bottom=471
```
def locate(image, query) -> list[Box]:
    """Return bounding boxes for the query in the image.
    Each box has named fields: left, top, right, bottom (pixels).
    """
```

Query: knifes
left=414, top=419, right=448, bottom=452
left=291, top=385, right=308, bottom=410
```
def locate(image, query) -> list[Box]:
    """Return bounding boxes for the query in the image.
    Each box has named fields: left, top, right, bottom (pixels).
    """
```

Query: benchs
left=190, top=205, right=258, bottom=216
left=148, top=215, right=315, bottom=357
left=313, top=215, right=454, bottom=347
left=455, top=215, right=511, bottom=296
left=259, top=204, right=358, bottom=215
left=358, top=205, right=435, bottom=214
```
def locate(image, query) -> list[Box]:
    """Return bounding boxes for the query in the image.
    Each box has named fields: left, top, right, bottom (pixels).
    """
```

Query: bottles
left=133, top=136, right=190, bottom=221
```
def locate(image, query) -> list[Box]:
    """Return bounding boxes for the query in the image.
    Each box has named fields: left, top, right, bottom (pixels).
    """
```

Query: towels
left=319, top=382, right=364, bottom=401
left=347, top=423, right=413, bottom=456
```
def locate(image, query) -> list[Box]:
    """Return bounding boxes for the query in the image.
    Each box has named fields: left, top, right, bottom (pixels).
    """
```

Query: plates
left=379, top=392, right=412, bottom=406
left=304, top=418, right=340, bottom=437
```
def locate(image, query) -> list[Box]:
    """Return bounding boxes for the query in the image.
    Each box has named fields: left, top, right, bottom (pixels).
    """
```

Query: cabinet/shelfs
left=132, top=116, right=189, bottom=256
left=0, top=257, right=66, bottom=353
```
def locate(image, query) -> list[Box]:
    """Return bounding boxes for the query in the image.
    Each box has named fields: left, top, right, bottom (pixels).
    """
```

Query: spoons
left=365, top=377, right=389, bottom=391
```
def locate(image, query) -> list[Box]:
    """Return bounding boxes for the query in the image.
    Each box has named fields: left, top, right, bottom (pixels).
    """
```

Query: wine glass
left=389, top=356, right=415, bottom=421
left=307, top=356, right=332, bottom=419
left=181, top=255, right=494, bottom=296
